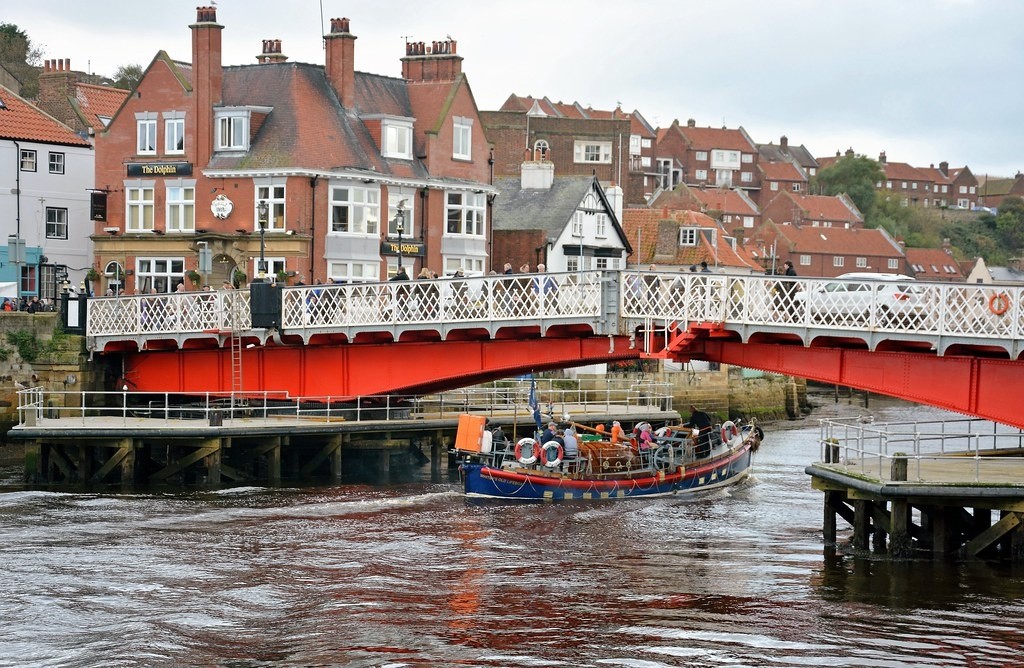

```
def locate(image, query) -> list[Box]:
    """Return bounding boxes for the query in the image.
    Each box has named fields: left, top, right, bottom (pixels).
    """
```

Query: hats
left=107, top=289, right=113, bottom=293
left=613, top=421, right=620, bottom=426
left=119, top=289, right=124, bottom=294
left=4, top=297, right=9, bottom=302
left=223, top=280, right=230, bottom=284
left=608, top=420, right=613, bottom=425
left=548, top=422, right=558, bottom=426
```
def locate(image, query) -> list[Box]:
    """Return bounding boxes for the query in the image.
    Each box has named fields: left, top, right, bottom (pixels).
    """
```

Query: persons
left=388, top=265, right=559, bottom=321
left=0, top=275, right=336, bottom=337
left=644, top=259, right=800, bottom=323
left=483, top=404, right=715, bottom=470
left=551, top=431, right=565, bottom=456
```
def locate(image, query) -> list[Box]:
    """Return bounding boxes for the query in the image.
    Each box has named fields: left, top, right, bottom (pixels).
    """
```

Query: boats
left=449, top=413, right=758, bottom=500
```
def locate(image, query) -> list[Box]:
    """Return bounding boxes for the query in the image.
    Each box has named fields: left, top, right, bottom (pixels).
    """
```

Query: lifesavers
left=654, top=427, right=672, bottom=444
left=989, top=294, right=1009, bottom=315
left=721, top=420, right=738, bottom=445
left=515, top=437, right=539, bottom=464
left=540, top=440, right=564, bottom=468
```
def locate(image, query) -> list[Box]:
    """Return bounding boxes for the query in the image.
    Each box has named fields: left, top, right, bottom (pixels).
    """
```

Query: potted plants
left=235, top=271, right=246, bottom=281
left=87, top=268, right=99, bottom=281
left=277, top=270, right=287, bottom=280
left=188, top=271, right=200, bottom=281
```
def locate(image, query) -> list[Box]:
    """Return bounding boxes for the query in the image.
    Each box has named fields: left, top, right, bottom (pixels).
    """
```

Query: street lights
left=256, top=200, right=269, bottom=278
left=394, top=209, right=405, bottom=268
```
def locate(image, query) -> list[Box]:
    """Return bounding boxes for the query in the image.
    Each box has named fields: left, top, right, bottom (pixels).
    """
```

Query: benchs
left=561, top=458, right=589, bottom=473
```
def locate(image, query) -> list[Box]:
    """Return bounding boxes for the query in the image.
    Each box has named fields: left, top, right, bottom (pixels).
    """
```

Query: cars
left=791, top=273, right=928, bottom=330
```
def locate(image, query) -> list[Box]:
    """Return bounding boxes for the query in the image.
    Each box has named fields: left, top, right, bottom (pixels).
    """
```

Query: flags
left=529, top=373, right=542, bottom=430
